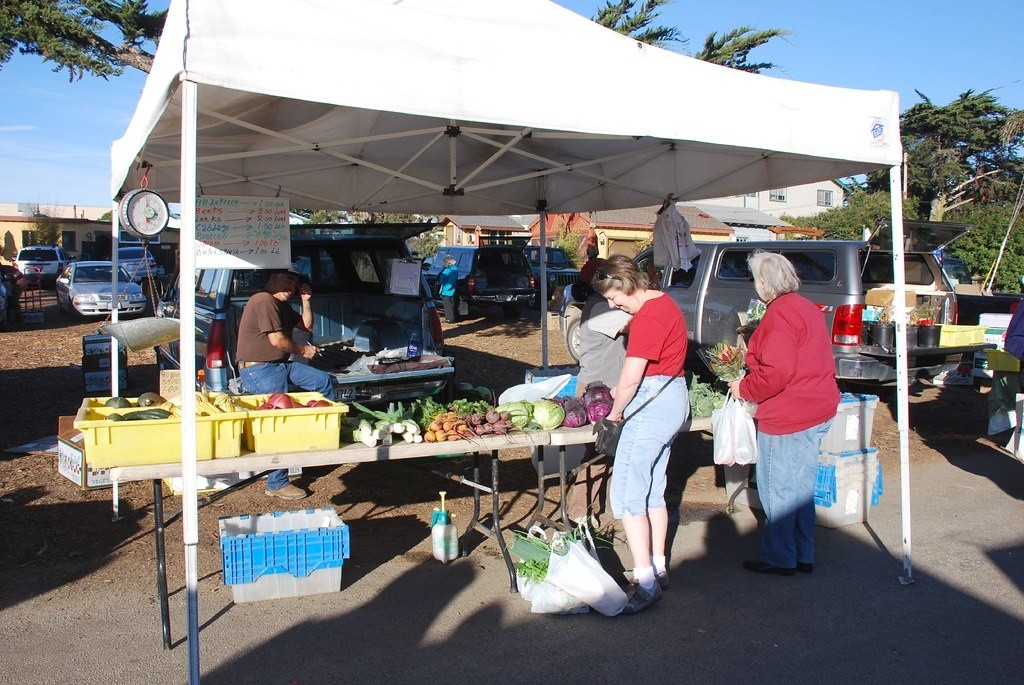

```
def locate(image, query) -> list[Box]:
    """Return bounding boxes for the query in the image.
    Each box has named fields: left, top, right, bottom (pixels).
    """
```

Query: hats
left=270, top=262, right=310, bottom=284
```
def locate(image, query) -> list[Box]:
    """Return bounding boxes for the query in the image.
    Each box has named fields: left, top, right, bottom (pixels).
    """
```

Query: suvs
left=12, top=244, right=75, bottom=288
left=423, top=246, right=536, bottom=320
left=523, top=245, right=580, bottom=308
left=154, top=224, right=456, bottom=405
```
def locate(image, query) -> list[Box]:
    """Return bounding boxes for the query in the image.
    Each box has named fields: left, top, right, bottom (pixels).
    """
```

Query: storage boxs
left=723, top=388, right=885, bottom=529
left=524, top=361, right=582, bottom=401
left=57, top=391, right=351, bottom=605
left=930, top=312, right=1020, bottom=385
left=219, top=506, right=352, bottom=604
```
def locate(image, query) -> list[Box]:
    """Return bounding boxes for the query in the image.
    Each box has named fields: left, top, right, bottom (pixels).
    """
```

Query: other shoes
left=442, top=320, right=456, bottom=324
left=742, top=562, right=815, bottom=575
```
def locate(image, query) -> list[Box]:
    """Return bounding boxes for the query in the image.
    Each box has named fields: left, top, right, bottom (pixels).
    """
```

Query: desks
left=522, top=391, right=727, bottom=543
left=110, top=417, right=548, bottom=650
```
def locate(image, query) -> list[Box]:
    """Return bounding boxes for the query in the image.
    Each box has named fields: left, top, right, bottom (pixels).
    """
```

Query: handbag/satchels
left=592, top=413, right=623, bottom=457
left=1006, top=393, right=1024, bottom=466
left=713, top=388, right=758, bottom=466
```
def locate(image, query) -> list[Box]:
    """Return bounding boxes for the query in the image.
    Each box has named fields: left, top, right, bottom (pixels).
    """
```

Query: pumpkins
left=105, top=392, right=234, bottom=422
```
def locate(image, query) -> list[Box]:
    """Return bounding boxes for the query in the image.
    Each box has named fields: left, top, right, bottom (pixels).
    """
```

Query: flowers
left=706, top=335, right=748, bottom=387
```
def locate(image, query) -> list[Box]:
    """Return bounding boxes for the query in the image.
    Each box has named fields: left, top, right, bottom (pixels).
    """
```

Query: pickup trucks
left=560, top=219, right=1024, bottom=398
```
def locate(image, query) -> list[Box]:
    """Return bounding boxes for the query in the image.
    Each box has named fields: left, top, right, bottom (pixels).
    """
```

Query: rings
left=306, top=287, right=308, bottom=289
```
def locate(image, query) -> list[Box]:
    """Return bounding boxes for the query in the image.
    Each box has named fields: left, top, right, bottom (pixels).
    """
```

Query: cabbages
left=495, top=399, right=565, bottom=429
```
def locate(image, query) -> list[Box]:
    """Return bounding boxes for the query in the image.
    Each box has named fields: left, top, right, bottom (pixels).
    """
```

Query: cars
left=56, top=260, right=146, bottom=320
left=117, top=247, right=157, bottom=283
left=0, top=262, right=20, bottom=329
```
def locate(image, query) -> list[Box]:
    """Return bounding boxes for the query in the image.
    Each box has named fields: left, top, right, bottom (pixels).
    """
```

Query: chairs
left=480, top=253, right=506, bottom=265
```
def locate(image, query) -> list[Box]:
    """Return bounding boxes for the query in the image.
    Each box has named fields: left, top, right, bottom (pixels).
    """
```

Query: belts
left=238, top=361, right=267, bottom=370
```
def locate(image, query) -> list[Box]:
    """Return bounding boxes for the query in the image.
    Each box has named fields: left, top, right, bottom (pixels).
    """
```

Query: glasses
left=596, top=269, right=622, bottom=282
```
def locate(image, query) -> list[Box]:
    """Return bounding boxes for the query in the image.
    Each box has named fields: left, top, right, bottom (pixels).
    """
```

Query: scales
left=100, top=187, right=204, bottom=353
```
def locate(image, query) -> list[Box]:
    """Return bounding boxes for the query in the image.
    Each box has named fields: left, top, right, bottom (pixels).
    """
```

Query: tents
left=106, top=1, right=913, bottom=683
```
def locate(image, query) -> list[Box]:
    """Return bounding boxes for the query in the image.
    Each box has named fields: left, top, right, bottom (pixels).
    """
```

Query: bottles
left=407, top=323, right=421, bottom=361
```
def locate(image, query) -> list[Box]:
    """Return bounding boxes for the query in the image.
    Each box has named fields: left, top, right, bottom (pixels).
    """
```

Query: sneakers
left=620, top=570, right=671, bottom=589
left=264, top=485, right=307, bottom=499
left=623, top=579, right=663, bottom=613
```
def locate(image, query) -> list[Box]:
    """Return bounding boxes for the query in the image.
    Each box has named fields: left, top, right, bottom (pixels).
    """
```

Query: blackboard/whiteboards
left=196, top=195, right=291, bottom=270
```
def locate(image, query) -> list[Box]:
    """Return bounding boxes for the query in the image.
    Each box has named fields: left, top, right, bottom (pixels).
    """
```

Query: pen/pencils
left=306, top=341, right=323, bottom=357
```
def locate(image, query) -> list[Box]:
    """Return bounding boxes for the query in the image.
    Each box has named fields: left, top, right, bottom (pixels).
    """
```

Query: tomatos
left=258, top=393, right=333, bottom=410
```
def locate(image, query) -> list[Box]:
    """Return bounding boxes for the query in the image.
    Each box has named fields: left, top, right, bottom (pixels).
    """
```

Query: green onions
left=340, top=401, right=422, bottom=447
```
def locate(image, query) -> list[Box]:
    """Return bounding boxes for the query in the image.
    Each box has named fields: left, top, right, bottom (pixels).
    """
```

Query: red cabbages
left=551, top=381, right=614, bottom=427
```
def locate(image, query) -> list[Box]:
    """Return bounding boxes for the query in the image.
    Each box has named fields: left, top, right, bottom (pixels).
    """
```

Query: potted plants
left=918, top=303, right=943, bottom=348
left=865, top=297, right=918, bottom=347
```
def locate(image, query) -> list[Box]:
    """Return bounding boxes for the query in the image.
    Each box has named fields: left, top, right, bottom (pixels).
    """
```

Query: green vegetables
left=513, top=502, right=622, bottom=581
left=684, top=370, right=726, bottom=417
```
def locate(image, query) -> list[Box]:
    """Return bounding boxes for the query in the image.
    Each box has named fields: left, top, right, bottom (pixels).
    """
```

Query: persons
left=0, top=265, right=28, bottom=326
left=566, top=257, right=663, bottom=530
left=727, top=252, right=841, bottom=577
left=237, top=263, right=334, bottom=499
left=438, top=255, right=458, bottom=323
left=592, top=256, right=689, bottom=615
